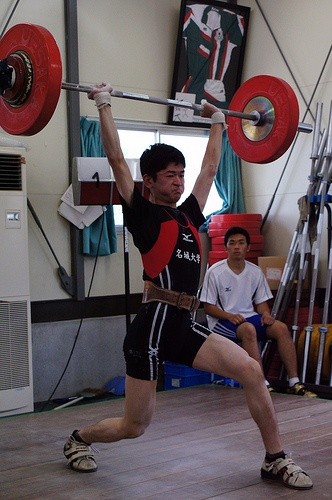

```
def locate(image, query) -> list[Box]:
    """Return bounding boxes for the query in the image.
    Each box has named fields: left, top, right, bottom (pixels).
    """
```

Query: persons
left=64, top=80, right=314, bottom=491
left=199, top=226, right=318, bottom=399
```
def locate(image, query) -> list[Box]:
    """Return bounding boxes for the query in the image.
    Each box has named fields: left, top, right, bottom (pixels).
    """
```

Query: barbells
left=0, top=24, right=313, bottom=164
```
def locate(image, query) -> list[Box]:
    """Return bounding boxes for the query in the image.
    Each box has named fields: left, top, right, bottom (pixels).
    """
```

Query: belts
left=142, top=280, right=200, bottom=312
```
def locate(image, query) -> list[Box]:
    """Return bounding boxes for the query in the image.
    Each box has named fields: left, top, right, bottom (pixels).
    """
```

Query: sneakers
left=63, top=429, right=101, bottom=473
left=260, top=452, right=313, bottom=490
left=283, top=382, right=319, bottom=398
left=266, top=384, right=277, bottom=393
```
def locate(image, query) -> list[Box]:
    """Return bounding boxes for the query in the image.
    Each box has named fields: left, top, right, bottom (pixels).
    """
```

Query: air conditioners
left=0, top=146, right=35, bottom=418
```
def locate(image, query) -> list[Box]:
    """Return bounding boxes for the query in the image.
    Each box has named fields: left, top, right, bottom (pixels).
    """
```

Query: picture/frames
left=168, top=0, right=251, bottom=129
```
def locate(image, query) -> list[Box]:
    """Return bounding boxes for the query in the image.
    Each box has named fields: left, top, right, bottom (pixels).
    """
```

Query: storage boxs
left=162, top=343, right=260, bottom=391
left=258, top=256, right=309, bottom=290
